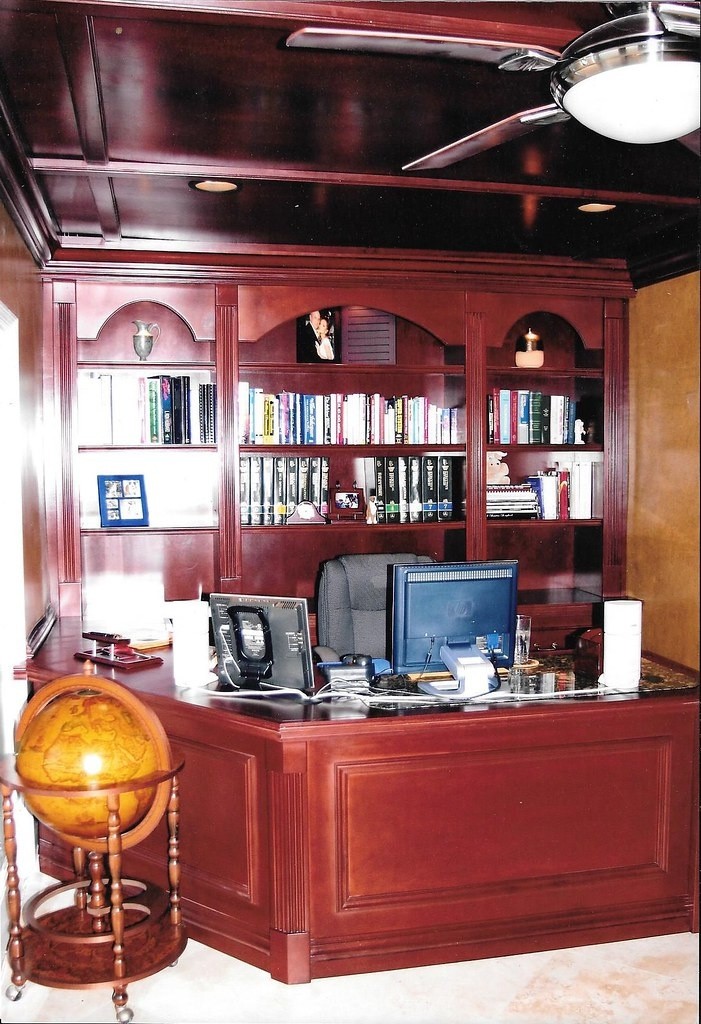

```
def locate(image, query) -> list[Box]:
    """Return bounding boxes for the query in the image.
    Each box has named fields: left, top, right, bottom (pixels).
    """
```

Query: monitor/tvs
left=390, top=560, right=519, bottom=700
left=209, top=592, right=315, bottom=699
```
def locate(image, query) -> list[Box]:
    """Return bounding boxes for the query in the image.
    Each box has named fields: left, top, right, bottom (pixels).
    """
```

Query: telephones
left=320, top=653, right=376, bottom=687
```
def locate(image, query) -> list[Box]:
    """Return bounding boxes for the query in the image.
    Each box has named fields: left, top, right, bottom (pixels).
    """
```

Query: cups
left=515, top=614, right=531, bottom=663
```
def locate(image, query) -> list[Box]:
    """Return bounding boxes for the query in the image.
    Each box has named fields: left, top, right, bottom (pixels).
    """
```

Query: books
left=487, top=461, right=604, bottom=520
left=364, top=457, right=453, bottom=522
left=137, top=375, right=217, bottom=444
left=487, top=387, right=575, bottom=444
left=28, top=606, right=56, bottom=655
left=237, top=382, right=466, bottom=445
left=128, top=627, right=171, bottom=649
left=239, top=457, right=329, bottom=525
left=98, top=475, right=149, bottom=527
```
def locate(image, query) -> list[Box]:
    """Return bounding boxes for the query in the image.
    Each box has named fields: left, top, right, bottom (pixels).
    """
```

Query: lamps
left=549, top=1, right=701, bottom=144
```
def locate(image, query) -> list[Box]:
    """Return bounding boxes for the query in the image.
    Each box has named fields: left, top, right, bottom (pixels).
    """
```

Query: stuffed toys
left=487, top=452, right=510, bottom=484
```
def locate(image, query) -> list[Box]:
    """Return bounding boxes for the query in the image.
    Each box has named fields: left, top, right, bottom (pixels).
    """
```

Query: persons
left=306, top=311, right=334, bottom=359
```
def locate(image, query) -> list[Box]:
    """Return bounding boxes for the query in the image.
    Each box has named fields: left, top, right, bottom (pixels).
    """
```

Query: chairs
left=312, top=553, right=436, bottom=665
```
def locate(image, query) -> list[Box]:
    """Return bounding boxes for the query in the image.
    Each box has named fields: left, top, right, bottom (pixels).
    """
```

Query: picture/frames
left=97, top=474, right=150, bottom=527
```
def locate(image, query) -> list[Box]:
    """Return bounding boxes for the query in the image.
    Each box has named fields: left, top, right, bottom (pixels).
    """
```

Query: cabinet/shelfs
left=37, top=248, right=638, bottom=633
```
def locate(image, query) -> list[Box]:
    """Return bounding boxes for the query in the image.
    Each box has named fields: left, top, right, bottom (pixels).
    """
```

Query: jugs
left=131, top=320, right=161, bottom=361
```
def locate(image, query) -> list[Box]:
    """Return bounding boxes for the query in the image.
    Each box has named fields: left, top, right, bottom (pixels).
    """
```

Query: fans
left=286, top=0, right=700, bottom=171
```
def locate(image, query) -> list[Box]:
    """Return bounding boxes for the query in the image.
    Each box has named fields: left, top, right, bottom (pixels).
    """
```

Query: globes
left=0, top=674, right=188, bottom=1024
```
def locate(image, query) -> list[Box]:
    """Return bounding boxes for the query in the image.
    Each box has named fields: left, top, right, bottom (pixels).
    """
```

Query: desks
left=28, top=645, right=701, bottom=985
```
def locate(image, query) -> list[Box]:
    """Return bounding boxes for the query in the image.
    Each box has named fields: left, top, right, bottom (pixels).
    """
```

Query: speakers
left=597, top=601, right=642, bottom=688
left=171, top=601, right=219, bottom=687
